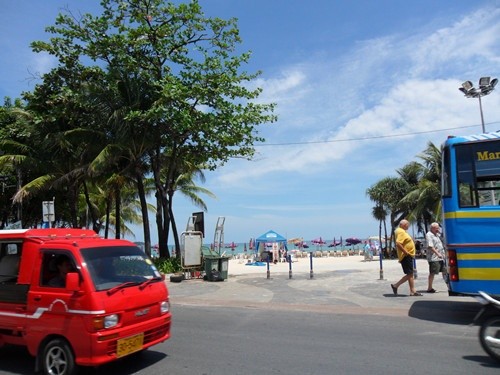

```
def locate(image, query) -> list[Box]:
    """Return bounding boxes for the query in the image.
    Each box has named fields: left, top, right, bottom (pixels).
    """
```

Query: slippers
left=391, top=284, right=398, bottom=296
left=427, top=288, right=436, bottom=292
left=410, top=292, right=423, bottom=296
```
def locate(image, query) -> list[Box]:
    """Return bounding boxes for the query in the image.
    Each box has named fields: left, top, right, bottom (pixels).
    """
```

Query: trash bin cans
left=204, top=256, right=229, bottom=281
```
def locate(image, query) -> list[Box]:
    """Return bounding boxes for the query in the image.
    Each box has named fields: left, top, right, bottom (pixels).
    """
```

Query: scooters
left=467, top=287, right=500, bottom=365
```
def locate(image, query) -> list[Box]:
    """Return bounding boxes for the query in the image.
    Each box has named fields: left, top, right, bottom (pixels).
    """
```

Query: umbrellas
left=152, top=238, right=253, bottom=254
left=288, top=236, right=362, bottom=251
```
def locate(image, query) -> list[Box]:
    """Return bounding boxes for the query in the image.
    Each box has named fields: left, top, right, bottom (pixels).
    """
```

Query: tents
left=255, top=230, right=287, bottom=261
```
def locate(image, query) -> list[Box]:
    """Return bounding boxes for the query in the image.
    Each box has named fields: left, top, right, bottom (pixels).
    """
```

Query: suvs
left=0, top=225, right=175, bottom=375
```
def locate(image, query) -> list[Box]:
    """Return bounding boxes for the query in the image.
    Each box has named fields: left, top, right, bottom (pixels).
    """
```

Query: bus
left=438, top=130, right=500, bottom=300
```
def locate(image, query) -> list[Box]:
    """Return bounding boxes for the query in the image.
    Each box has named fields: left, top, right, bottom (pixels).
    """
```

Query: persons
left=425, top=222, right=449, bottom=293
left=382, top=247, right=398, bottom=259
left=363, top=239, right=375, bottom=261
left=391, top=219, right=423, bottom=296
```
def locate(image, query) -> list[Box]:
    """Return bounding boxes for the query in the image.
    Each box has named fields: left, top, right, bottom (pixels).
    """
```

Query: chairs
left=295, top=250, right=364, bottom=258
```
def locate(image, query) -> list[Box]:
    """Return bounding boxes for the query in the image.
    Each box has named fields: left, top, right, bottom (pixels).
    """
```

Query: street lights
left=458, top=76, right=499, bottom=135
